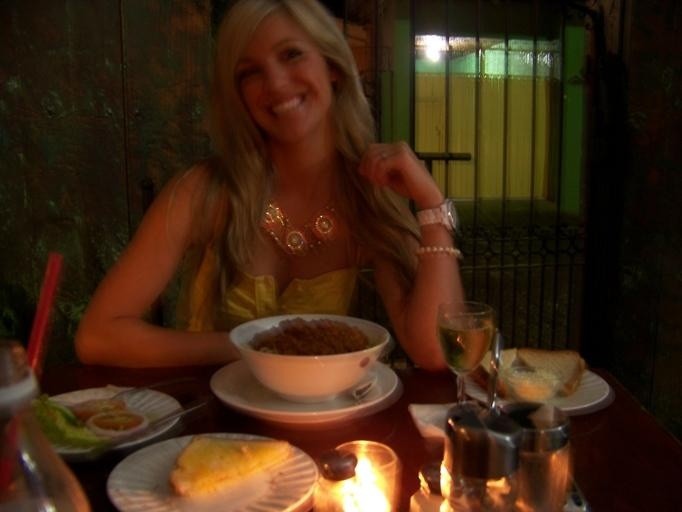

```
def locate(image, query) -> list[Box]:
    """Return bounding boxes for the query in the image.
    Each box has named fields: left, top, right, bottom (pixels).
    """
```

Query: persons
left=73, top=0, right=467, bottom=376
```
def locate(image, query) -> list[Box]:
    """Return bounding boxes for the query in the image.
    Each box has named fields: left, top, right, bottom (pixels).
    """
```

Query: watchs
left=414, top=196, right=460, bottom=239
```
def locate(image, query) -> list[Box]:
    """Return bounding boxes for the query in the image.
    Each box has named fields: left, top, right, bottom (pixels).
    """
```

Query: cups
left=336, top=440, right=400, bottom=512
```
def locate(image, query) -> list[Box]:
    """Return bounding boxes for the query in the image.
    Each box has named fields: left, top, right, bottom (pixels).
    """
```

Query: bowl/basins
left=228, top=312, right=392, bottom=406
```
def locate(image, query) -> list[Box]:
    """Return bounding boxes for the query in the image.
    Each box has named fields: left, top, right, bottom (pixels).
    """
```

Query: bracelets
left=412, top=242, right=466, bottom=264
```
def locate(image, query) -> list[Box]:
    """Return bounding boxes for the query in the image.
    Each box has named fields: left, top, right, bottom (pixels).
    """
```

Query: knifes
left=87, top=398, right=207, bottom=461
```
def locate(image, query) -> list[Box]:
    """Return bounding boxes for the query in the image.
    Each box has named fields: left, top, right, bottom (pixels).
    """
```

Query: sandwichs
left=481, top=346, right=587, bottom=394
left=169, top=430, right=293, bottom=501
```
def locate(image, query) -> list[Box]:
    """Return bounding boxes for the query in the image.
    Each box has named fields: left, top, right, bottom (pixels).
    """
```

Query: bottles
left=440, top=401, right=572, bottom=511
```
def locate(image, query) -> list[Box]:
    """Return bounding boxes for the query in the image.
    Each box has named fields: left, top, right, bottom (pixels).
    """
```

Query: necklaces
left=260, top=197, right=341, bottom=256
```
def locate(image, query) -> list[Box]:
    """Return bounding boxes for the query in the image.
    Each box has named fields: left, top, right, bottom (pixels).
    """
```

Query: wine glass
left=436, top=300, right=497, bottom=402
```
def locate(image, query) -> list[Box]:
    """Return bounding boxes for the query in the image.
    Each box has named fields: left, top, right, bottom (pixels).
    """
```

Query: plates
left=41, top=384, right=183, bottom=458
left=106, top=431, right=320, bottom=512
left=463, top=365, right=611, bottom=413
left=209, top=358, right=399, bottom=432
